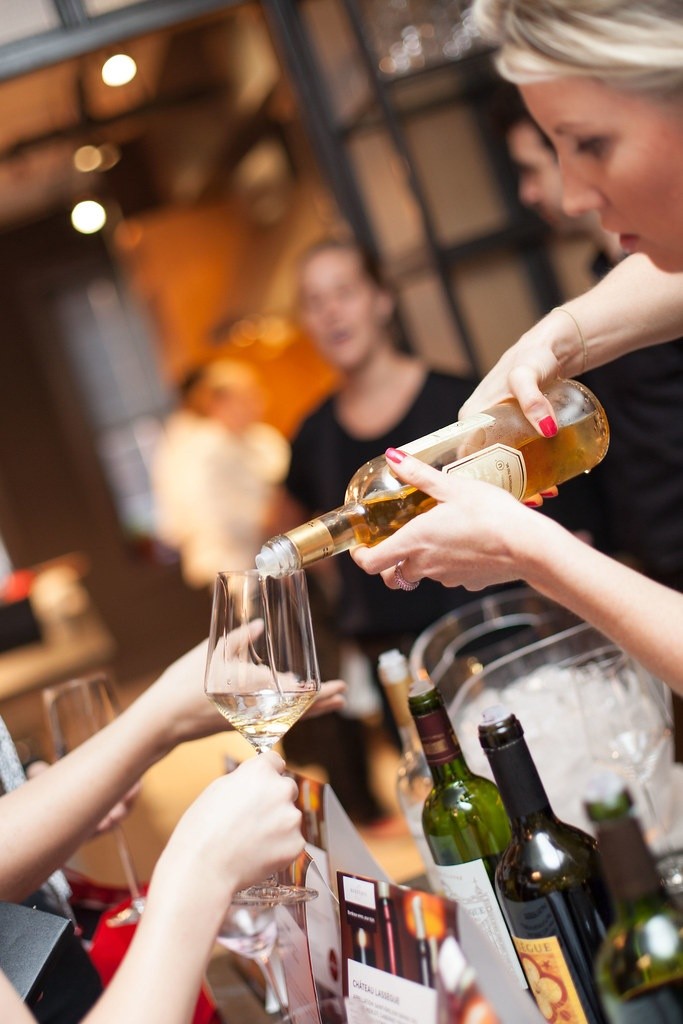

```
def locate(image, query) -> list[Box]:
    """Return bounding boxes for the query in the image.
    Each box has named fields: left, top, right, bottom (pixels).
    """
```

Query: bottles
left=378, top=650, right=434, bottom=898
left=378, top=882, right=403, bottom=978
left=412, top=895, right=434, bottom=988
left=255, top=378, right=610, bottom=579
left=585, top=780, right=683, bottom=1024
left=479, top=704, right=615, bottom=1024
left=408, top=679, right=513, bottom=1004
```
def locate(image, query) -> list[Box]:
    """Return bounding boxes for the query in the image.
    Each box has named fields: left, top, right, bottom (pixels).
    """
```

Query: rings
left=394, top=561, right=419, bottom=591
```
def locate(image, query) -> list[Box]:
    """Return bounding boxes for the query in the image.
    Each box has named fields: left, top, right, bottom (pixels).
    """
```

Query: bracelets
left=550, top=307, right=587, bottom=373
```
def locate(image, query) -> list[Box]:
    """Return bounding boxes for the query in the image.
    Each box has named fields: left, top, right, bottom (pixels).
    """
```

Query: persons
left=495, top=94, right=682, bottom=601
left=155, top=241, right=481, bottom=650
left=0, top=616, right=346, bottom=1024
left=350, top=0, right=683, bottom=699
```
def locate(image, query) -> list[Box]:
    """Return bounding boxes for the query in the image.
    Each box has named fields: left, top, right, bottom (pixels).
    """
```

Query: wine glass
left=216, top=906, right=292, bottom=1024
left=43, top=672, right=147, bottom=926
left=204, top=569, right=321, bottom=906
